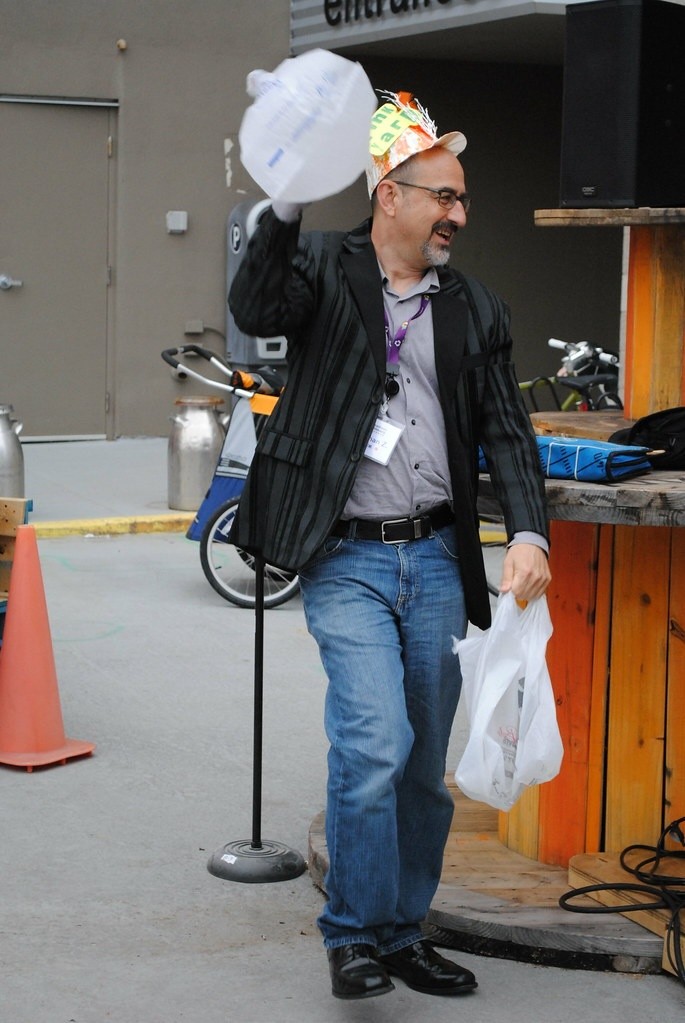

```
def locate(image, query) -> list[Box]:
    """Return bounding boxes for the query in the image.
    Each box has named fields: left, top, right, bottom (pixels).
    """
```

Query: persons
left=228, top=98, right=554, bottom=1003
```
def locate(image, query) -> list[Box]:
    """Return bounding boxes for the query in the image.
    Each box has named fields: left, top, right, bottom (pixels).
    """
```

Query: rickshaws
left=161, top=343, right=510, bottom=609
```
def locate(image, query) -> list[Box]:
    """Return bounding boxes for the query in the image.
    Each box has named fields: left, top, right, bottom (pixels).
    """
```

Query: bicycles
left=459, top=336, right=620, bottom=599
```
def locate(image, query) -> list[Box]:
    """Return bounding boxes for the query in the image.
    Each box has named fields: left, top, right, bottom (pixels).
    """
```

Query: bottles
left=0, top=404, right=28, bottom=504
left=161, top=396, right=231, bottom=511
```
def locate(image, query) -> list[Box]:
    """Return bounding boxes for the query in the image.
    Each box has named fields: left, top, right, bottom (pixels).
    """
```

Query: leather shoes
left=373, top=942, right=479, bottom=996
left=327, top=938, right=394, bottom=1001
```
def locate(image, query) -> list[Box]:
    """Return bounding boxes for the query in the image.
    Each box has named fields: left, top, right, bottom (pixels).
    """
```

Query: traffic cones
left=1, top=525, right=94, bottom=772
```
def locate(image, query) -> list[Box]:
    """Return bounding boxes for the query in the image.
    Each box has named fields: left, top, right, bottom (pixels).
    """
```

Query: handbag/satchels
left=239, top=48, right=378, bottom=207
left=604, top=409, right=685, bottom=473
left=473, top=428, right=663, bottom=482
